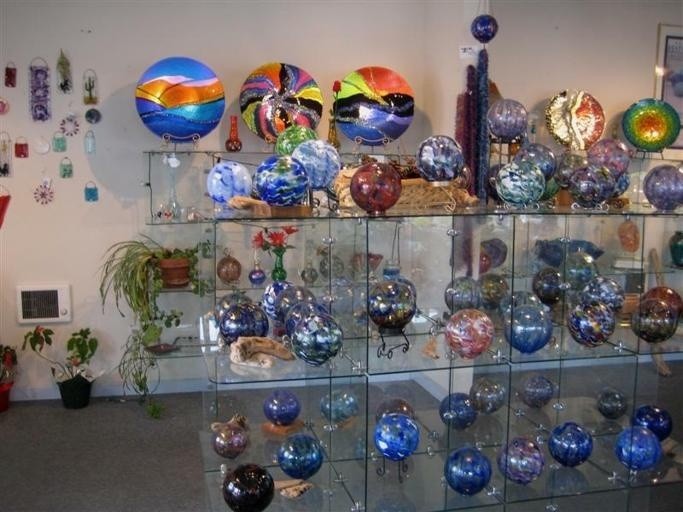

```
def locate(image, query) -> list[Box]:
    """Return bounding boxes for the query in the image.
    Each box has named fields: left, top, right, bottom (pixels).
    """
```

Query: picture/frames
left=651, top=22, right=683, bottom=148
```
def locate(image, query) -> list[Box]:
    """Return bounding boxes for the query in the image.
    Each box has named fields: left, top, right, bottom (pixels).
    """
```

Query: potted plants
left=116, top=239, right=206, bottom=416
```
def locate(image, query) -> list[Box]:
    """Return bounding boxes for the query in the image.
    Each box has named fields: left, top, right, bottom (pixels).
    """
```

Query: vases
left=0, top=379, right=93, bottom=417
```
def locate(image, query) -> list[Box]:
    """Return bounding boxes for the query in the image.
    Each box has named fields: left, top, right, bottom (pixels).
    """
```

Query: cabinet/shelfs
left=146, top=149, right=683, bottom=510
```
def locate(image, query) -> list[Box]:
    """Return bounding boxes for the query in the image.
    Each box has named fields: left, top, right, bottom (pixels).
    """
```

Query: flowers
left=0, top=328, right=100, bottom=384
